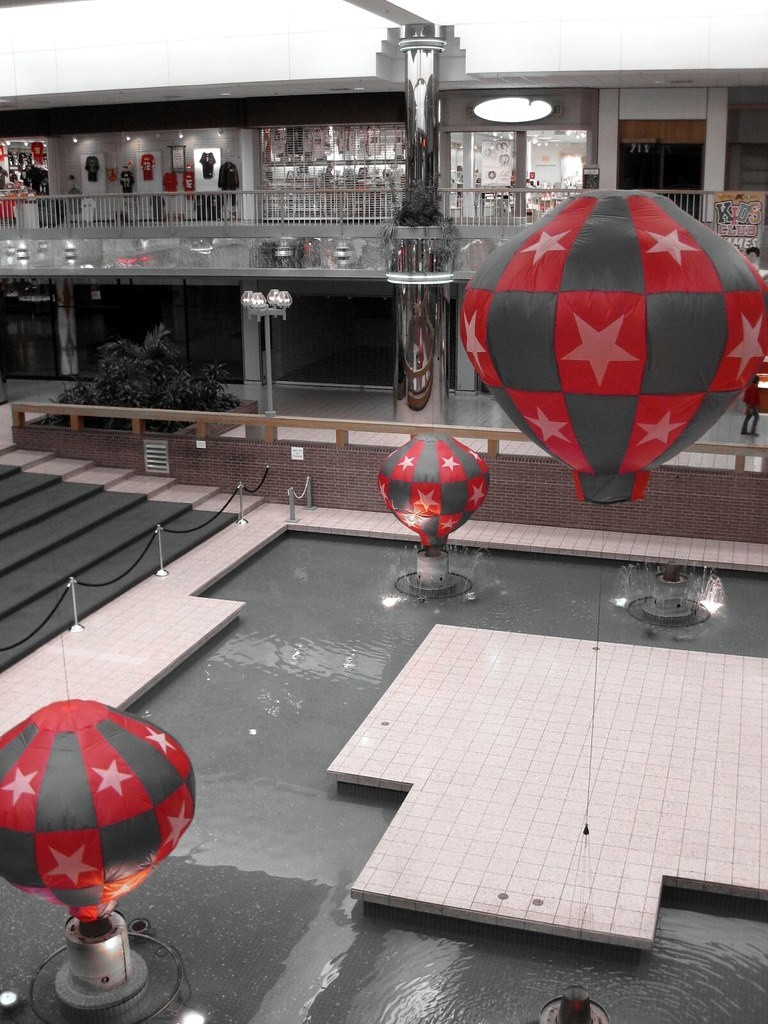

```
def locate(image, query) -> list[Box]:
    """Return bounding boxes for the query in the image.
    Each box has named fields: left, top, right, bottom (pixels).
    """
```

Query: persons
left=741, top=375, right=761, bottom=437
